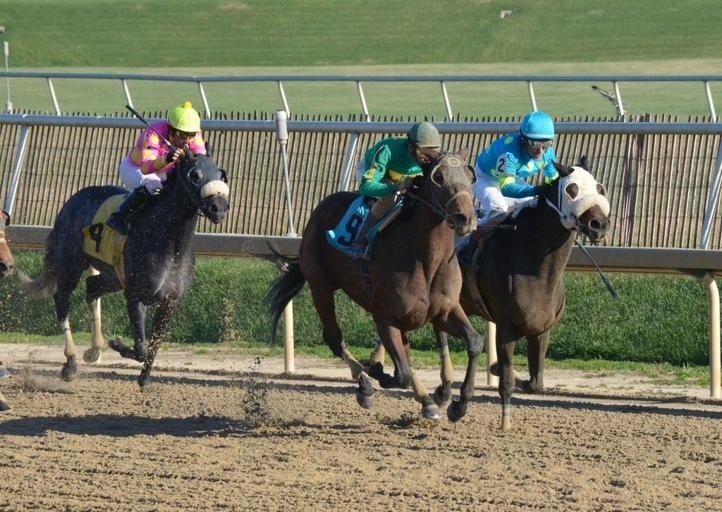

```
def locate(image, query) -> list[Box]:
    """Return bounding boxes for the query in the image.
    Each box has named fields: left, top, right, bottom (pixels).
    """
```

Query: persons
left=344, top=122, right=440, bottom=259
left=471, top=109, right=559, bottom=225
left=106, top=102, right=206, bottom=235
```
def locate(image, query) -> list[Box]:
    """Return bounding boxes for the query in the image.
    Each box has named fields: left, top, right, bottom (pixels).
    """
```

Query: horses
left=265, top=146, right=481, bottom=422
left=14, top=142, right=232, bottom=395
left=370, top=155, right=608, bottom=432
left=0, top=210, right=15, bottom=411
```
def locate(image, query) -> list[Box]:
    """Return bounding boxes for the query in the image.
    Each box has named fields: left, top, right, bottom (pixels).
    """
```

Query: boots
left=346, top=211, right=382, bottom=256
left=106, top=185, right=155, bottom=235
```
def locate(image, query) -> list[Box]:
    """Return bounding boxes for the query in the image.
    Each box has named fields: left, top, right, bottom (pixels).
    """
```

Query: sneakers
left=458, top=238, right=478, bottom=269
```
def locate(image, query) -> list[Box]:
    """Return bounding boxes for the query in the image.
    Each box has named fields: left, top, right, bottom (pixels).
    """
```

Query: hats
left=168, top=100, right=201, bottom=133
left=519, top=110, right=555, bottom=140
left=408, top=121, right=441, bottom=148
